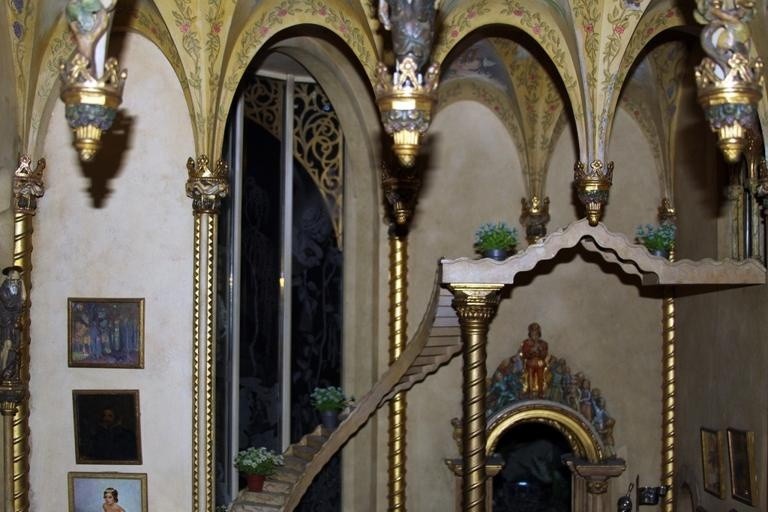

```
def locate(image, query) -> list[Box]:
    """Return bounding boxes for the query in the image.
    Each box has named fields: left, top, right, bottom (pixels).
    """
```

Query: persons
left=101, top=487, right=124, bottom=512
left=521, top=322, right=548, bottom=401
left=71, top=303, right=140, bottom=361
left=0, top=264, right=28, bottom=381
left=96, top=406, right=137, bottom=460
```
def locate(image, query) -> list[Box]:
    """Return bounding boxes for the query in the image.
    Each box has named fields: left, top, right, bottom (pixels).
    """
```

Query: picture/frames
left=699, top=424, right=724, bottom=500
left=66, top=295, right=144, bottom=369
left=726, top=424, right=758, bottom=507
left=66, top=472, right=149, bottom=511
left=71, top=388, right=143, bottom=467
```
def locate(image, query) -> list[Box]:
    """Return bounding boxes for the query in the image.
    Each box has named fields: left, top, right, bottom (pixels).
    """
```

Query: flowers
left=310, top=386, right=356, bottom=410
left=233, top=446, right=284, bottom=477
left=635, top=222, right=678, bottom=251
left=476, top=219, right=521, bottom=252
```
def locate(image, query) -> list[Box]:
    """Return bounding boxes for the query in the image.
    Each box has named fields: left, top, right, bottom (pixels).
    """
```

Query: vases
left=484, top=249, right=506, bottom=260
left=651, top=249, right=668, bottom=260
left=321, top=410, right=337, bottom=428
left=246, top=475, right=264, bottom=492
